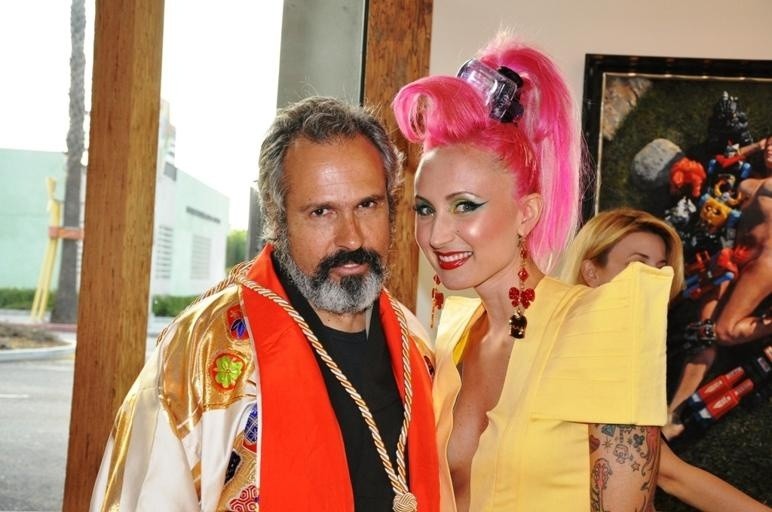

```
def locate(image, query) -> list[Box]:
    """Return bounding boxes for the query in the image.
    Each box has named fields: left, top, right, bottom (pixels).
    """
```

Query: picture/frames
left=579, top=54, right=772, bottom=512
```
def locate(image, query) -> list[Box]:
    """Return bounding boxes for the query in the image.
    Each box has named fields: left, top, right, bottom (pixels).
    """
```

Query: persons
left=560, top=208, right=772, bottom=511
left=393, top=35, right=676, bottom=512
left=88, top=97, right=438, bottom=512
left=668, top=134, right=772, bottom=423
